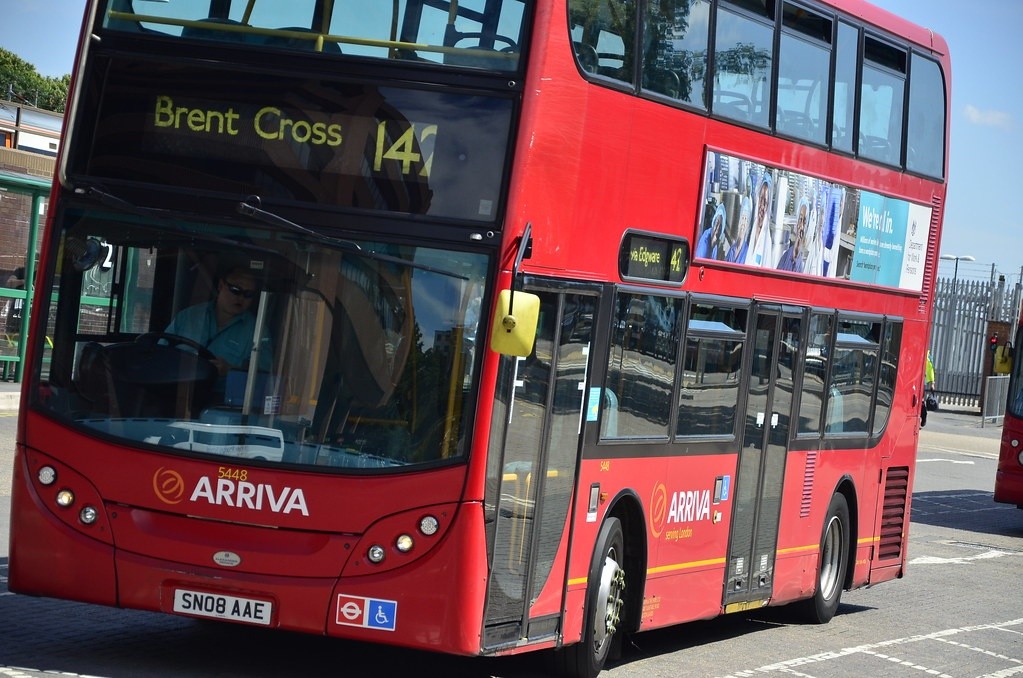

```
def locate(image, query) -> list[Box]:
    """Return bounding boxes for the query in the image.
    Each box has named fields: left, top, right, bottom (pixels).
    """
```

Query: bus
left=7, top=0, right=951, bottom=678
left=993, top=300, right=1023, bottom=510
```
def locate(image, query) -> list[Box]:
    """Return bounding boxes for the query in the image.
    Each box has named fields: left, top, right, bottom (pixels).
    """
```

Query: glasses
left=222, top=278, right=257, bottom=299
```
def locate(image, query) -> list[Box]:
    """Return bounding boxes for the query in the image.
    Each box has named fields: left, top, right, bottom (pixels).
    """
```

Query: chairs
left=826, top=389, right=843, bottom=433
left=182, top=18, right=342, bottom=55
left=445, top=33, right=916, bottom=170
left=601, top=388, right=619, bottom=438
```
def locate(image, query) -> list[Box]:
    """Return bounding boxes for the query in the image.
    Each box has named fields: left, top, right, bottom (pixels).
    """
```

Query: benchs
left=0, top=356, right=20, bottom=381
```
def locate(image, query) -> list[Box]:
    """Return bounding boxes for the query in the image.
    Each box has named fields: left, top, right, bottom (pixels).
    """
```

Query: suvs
left=622, top=297, right=645, bottom=351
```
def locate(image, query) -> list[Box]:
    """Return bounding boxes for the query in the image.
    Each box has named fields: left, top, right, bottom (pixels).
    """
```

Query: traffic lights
left=989, top=337, right=998, bottom=353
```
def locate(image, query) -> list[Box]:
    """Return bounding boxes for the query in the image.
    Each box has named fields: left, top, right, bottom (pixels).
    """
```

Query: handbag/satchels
left=926, top=390, right=937, bottom=411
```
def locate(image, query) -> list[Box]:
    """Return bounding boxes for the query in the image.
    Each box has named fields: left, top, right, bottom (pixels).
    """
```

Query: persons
left=696, top=173, right=826, bottom=279
left=920, top=350, right=935, bottom=428
left=158, top=262, right=274, bottom=415
left=6, top=252, right=40, bottom=351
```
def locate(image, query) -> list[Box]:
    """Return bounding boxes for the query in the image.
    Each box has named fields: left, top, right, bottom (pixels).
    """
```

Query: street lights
left=940, top=255, right=976, bottom=297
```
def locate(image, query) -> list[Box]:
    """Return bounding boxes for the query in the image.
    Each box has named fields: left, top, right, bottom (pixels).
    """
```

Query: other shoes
left=921, top=417, right=926, bottom=427
